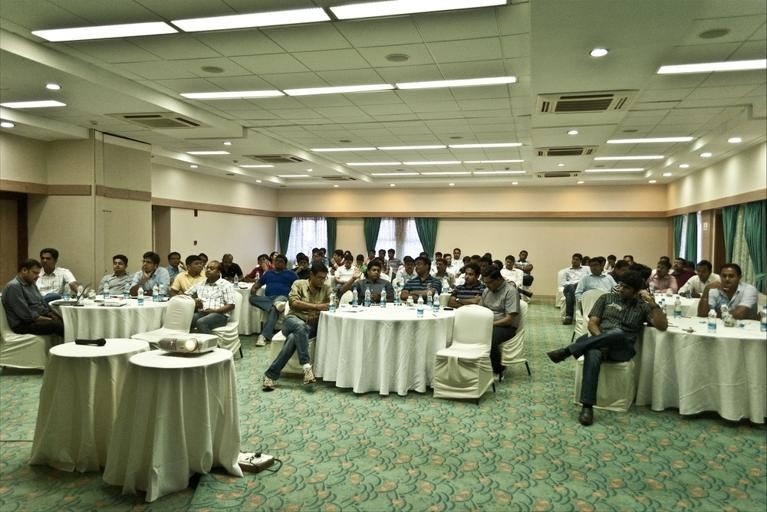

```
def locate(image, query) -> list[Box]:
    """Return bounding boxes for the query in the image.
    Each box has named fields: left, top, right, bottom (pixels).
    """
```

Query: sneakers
left=303, top=368, right=316, bottom=384
left=563, top=317, right=572, bottom=325
left=256, top=335, right=266, bottom=347
left=262, top=375, right=276, bottom=392
left=275, top=301, right=286, bottom=312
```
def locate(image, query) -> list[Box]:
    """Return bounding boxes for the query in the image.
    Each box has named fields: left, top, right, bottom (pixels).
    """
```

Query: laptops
left=51, top=284, right=91, bottom=306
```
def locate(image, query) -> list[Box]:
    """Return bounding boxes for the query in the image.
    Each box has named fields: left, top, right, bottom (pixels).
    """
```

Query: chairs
left=205, top=289, right=246, bottom=361
left=579, top=284, right=609, bottom=319
left=125, top=286, right=199, bottom=342
left=0, top=287, right=63, bottom=376
left=271, top=301, right=314, bottom=384
left=492, top=297, right=534, bottom=382
left=433, top=301, right=503, bottom=405
left=572, top=313, right=638, bottom=415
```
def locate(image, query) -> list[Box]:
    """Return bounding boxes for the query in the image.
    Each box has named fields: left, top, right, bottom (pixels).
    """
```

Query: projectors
left=158, top=333, right=219, bottom=355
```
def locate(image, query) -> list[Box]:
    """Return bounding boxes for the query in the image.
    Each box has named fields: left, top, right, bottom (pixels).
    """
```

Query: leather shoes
left=547, top=347, right=570, bottom=363
left=579, top=405, right=594, bottom=427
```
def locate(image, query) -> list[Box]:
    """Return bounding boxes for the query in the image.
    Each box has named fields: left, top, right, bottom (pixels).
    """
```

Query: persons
left=2, top=248, right=80, bottom=337
left=96, top=251, right=245, bottom=347
left=242, top=248, right=533, bottom=391
left=547, top=253, right=758, bottom=426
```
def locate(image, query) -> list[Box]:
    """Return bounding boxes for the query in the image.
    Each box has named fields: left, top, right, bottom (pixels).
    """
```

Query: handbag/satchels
left=236, top=451, right=282, bottom=475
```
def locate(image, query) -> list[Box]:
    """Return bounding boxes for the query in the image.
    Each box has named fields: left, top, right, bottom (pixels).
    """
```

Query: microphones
left=75, top=338, right=106, bottom=346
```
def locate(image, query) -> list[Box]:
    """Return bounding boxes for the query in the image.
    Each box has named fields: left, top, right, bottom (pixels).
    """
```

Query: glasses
left=317, top=276, right=328, bottom=281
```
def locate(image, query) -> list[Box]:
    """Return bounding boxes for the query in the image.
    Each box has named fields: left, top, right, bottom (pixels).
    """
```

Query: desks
left=30, top=340, right=150, bottom=477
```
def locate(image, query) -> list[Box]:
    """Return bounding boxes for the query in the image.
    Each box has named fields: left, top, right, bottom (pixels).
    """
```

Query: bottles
left=330, top=290, right=336, bottom=312
left=125, top=282, right=131, bottom=295
left=364, top=286, right=372, bottom=307
left=381, top=286, right=387, bottom=307
left=353, top=287, right=360, bottom=307
left=64, top=281, right=70, bottom=299
left=152, top=282, right=159, bottom=301
left=427, top=288, right=432, bottom=306
left=760, top=305, right=766, bottom=331
left=418, top=294, right=424, bottom=317
left=138, top=285, right=144, bottom=306
left=674, top=297, right=682, bottom=320
left=103, top=280, right=109, bottom=298
left=394, top=286, right=401, bottom=305
left=234, top=273, right=239, bottom=288
left=434, top=292, right=441, bottom=312
left=662, top=299, right=666, bottom=314
left=708, top=306, right=717, bottom=332
